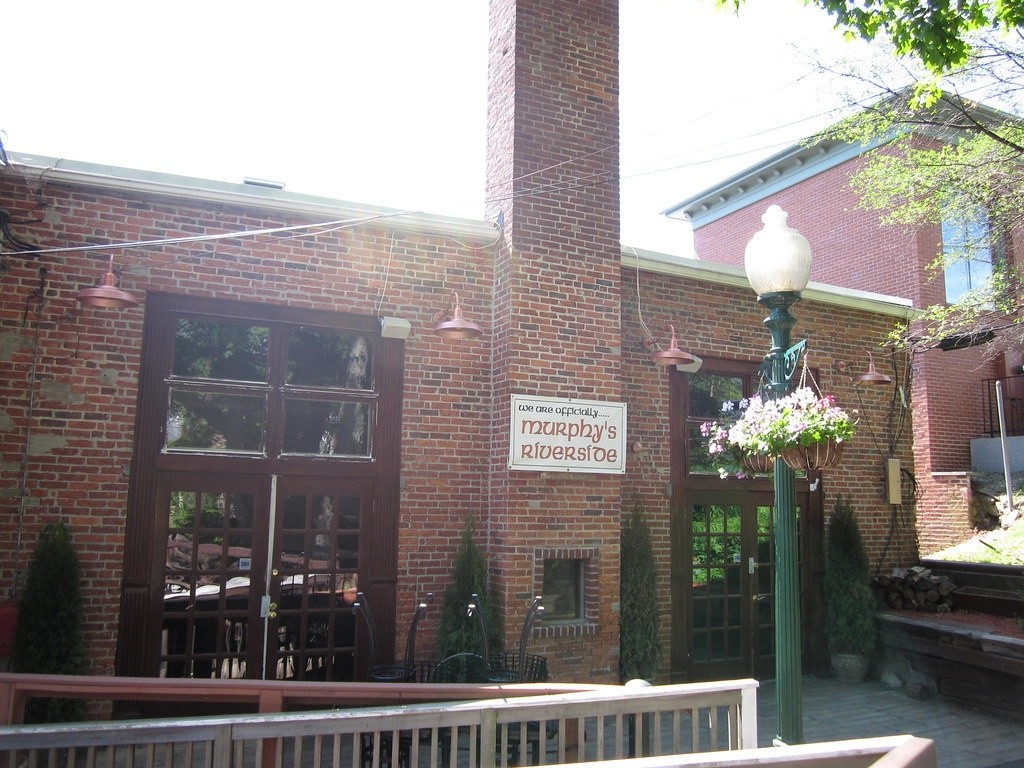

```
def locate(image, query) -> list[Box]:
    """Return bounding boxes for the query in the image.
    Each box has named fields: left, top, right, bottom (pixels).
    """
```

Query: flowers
left=818, top=439, right=849, bottom=439
left=699, top=399, right=773, bottom=483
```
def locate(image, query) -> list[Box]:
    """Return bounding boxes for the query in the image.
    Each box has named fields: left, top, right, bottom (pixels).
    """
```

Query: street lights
left=745, top=202, right=816, bottom=748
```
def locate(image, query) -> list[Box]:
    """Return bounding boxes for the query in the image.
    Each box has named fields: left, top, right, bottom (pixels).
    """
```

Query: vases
left=763, top=382, right=867, bottom=450
left=777, top=441, right=847, bottom=473
left=742, top=451, right=774, bottom=478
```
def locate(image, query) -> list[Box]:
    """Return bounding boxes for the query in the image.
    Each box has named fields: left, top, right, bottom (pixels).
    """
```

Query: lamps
left=643, top=319, right=698, bottom=373
left=838, top=350, right=895, bottom=393
left=430, top=282, right=485, bottom=346
left=70, top=251, right=142, bottom=323
